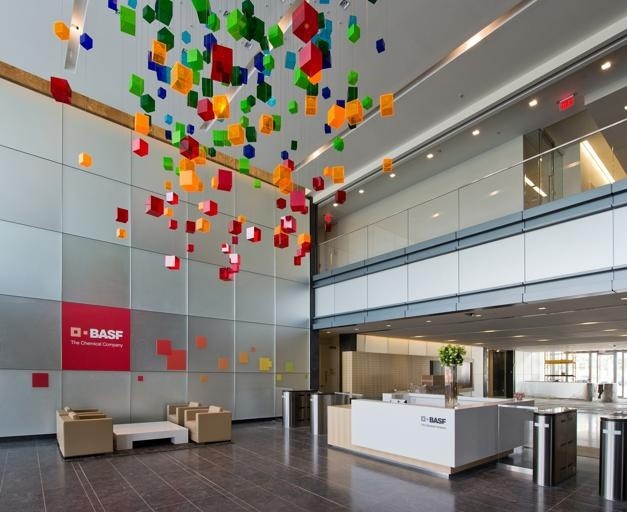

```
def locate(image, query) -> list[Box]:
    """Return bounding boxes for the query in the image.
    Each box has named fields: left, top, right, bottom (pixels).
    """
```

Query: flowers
left=437, top=342, right=466, bottom=368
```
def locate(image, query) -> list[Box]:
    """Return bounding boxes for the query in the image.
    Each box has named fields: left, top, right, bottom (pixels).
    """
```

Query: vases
left=444, top=364, right=458, bottom=409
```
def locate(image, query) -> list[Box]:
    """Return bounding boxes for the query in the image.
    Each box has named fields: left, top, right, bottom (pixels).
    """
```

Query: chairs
left=166, top=401, right=232, bottom=444
left=55, top=405, right=113, bottom=459
